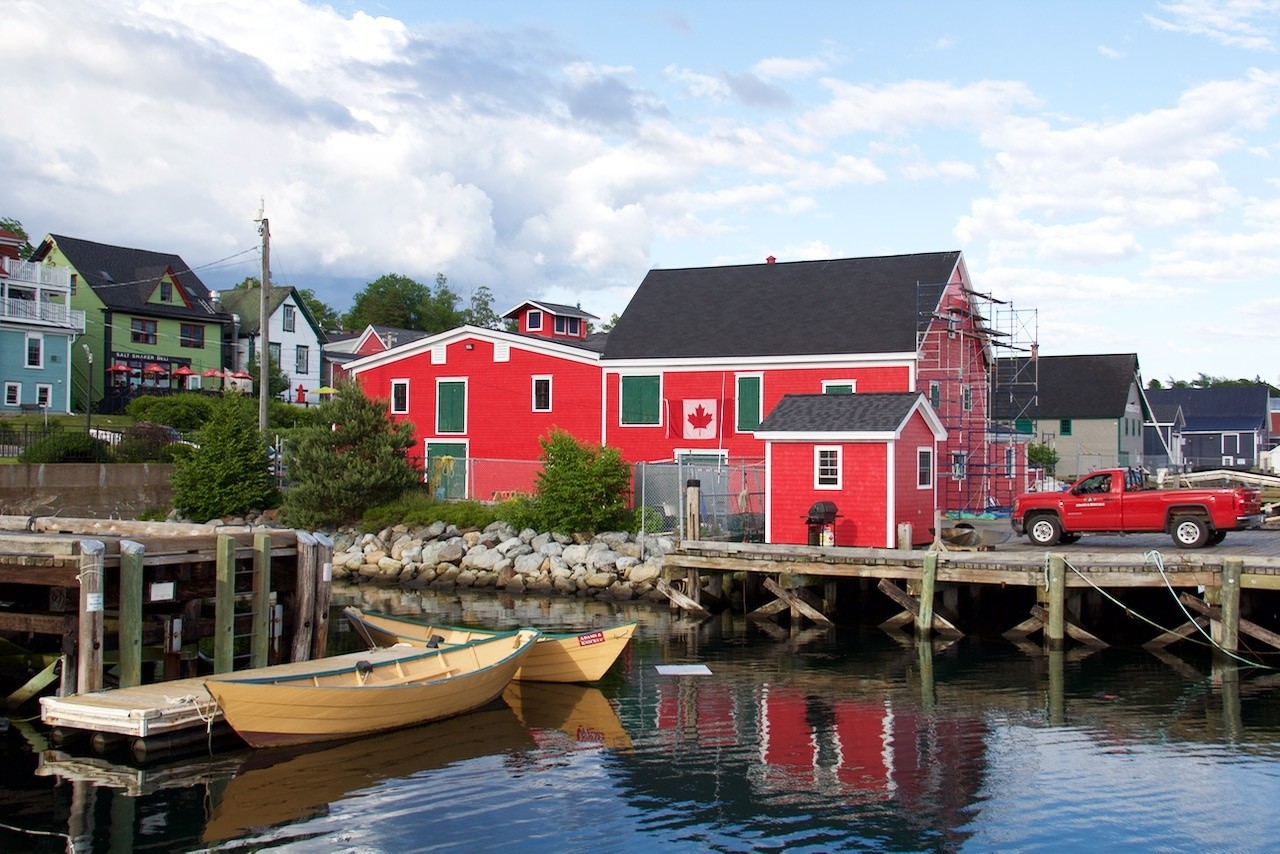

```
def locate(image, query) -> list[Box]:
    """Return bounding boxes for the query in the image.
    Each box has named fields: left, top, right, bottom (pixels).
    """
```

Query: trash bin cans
left=805, top=502, right=839, bottom=546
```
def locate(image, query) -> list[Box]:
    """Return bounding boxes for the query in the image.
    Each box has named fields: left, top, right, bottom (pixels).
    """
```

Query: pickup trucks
left=89, top=422, right=180, bottom=451
left=1010, top=467, right=1264, bottom=550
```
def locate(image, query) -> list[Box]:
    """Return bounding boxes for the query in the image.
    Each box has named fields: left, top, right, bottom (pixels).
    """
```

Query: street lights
left=82, top=343, right=93, bottom=439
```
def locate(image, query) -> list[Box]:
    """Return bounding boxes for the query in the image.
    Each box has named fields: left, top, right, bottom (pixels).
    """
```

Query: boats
left=199, top=695, right=540, bottom=835
left=497, top=681, right=632, bottom=752
left=202, top=624, right=545, bottom=748
left=341, top=603, right=638, bottom=686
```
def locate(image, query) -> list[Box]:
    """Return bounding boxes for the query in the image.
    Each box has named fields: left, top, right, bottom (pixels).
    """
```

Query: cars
left=267, top=446, right=299, bottom=482
